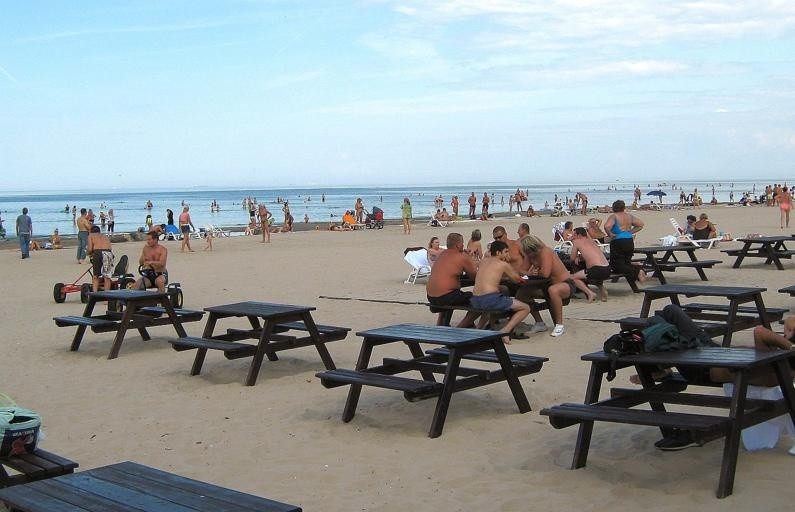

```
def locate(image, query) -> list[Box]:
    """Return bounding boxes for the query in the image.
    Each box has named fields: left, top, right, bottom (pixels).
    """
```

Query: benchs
left=54, top=290, right=353, bottom=386
left=316, top=234, right=795, bottom=498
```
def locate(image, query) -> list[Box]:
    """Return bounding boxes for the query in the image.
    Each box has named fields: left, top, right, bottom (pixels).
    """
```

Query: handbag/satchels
left=663, top=235, right=677, bottom=246
left=604, top=329, right=642, bottom=356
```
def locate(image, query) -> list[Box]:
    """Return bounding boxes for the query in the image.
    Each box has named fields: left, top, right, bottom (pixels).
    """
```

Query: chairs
left=582, top=221, right=611, bottom=255
left=165, top=209, right=454, bottom=242
left=669, top=218, right=722, bottom=249
left=552, top=222, right=582, bottom=255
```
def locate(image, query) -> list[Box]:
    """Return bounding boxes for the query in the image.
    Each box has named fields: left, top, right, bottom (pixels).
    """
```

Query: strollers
left=361, top=206, right=385, bottom=229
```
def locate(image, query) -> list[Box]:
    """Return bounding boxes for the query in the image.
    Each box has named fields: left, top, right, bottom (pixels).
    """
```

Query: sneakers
left=551, top=323, right=565, bottom=336
left=654, top=428, right=702, bottom=450
left=529, top=325, right=548, bottom=332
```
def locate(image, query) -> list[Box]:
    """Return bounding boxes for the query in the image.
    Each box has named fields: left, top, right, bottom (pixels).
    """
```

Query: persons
left=146, top=194, right=325, bottom=252
left=400, top=198, right=413, bottom=234
left=28, top=229, right=63, bottom=251
left=132, top=231, right=168, bottom=292
left=678, top=188, right=765, bottom=209
left=85, top=226, right=116, bottom=305
left=434, top=188, right=541, bottom=221
left=342, top=198, right=364, bottom=230
left=65, top=204, right=114, bottom=264
left=551, top=190, right=588, bottom=217
left=427, top=222, right=577, bottom=345
left=629, top=305, right=794, bottom=387
left=764, top=184, right=795, bottom=229
left=16, top=208, right=32, bottom=259
left=684, top=213, right=719, bottom=247
left=658, top=190, right=662, bottom=203
left=564, top=200, right=647, bottom=304
left=633, top=187, right=641, bottom=206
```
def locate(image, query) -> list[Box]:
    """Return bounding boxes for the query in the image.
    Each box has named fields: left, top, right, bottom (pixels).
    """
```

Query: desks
left=0, top=464, right=307, bottom=512
